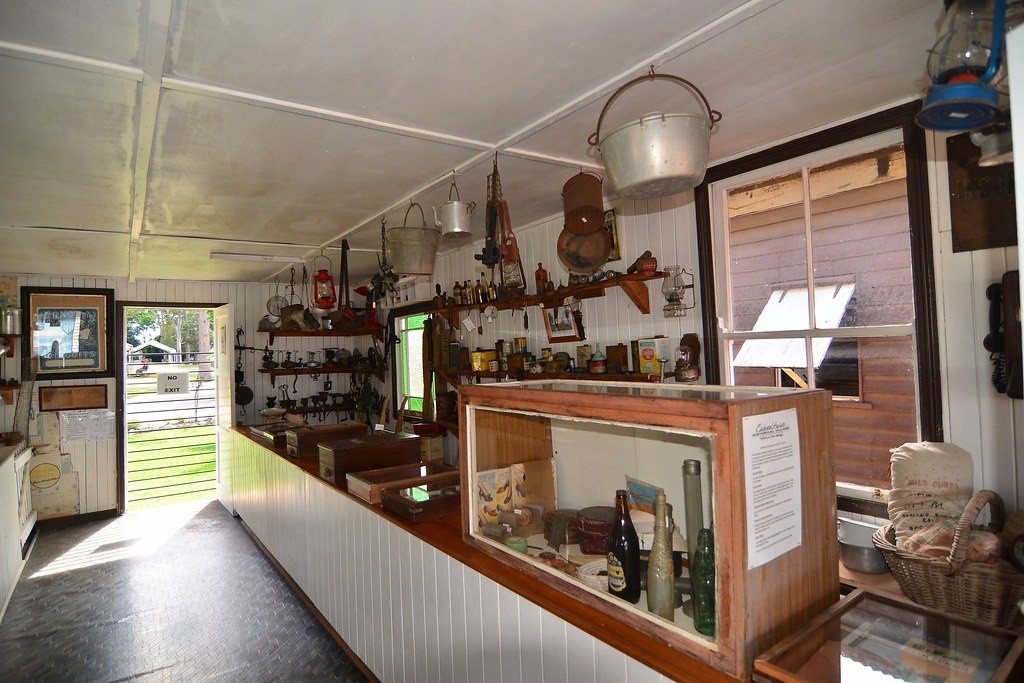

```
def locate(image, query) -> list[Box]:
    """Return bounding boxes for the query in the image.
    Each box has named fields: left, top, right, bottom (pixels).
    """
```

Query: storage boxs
left=285, top=418, right=459, bottom=524
left=457, top=379, right=836, bottom=677
left=638, top=337, right=670, bottom=374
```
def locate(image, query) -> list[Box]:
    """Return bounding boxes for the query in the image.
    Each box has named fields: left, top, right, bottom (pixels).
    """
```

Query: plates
left=558, top=225, right=612, bottom=273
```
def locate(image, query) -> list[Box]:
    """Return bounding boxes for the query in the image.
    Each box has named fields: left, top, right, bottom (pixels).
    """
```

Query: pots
left=0, top=304, right=23, bottom=334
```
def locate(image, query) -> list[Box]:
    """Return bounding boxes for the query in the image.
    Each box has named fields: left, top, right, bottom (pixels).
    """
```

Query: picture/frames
left=39, top=384, right=108, bottom=411
left=20, top=285, right=116, bottom=381
left=565, top=210, right=622, bottom=273
left=542, top=304, right=581, bottom=343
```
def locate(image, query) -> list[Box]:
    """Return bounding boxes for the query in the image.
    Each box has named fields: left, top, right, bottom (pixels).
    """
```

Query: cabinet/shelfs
left=257, top=327, right=383, bottom=414
left=425, top=272, right=674, bottom=383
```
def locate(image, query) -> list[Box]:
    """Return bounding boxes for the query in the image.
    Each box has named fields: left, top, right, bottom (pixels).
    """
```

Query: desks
left=750, top=588, right=1024, bottom=683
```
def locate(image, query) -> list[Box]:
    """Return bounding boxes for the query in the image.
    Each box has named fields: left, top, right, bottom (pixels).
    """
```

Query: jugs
left=431, top=183, right=477, bottom=239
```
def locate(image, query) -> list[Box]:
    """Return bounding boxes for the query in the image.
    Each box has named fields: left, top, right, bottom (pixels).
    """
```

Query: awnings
left=733, top=282, right=854, bottom=388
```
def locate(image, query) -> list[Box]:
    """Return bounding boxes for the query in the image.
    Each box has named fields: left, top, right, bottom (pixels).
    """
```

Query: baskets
left=871, top=489, right=1024, bottom=635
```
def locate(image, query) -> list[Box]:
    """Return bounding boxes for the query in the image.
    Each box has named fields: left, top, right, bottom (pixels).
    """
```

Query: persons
left=136, top=359, right=148, bottom=377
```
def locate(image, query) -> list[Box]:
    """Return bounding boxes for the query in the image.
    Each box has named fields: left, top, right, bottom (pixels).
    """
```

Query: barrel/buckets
left=513, top=337, right=528, bottom=354
left=561, top=171, right=608, bottom=235
left=385, top=203, right=440, bottom=276
left=588, top=73, right=722, bottom=199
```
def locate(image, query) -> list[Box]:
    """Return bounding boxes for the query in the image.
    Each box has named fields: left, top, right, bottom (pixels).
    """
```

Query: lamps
left=915, top=0, right=1024, bottom=134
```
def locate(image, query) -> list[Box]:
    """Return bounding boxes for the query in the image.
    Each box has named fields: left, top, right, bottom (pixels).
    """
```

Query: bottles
left=534, top=261, right=548, bottom=294
left=453, top=272, right=501, bottom=306
left=592, top=343, right=607, bottom=374
left=691, top=529, right=715, bottom=636
left=607, top=490, right=641, bottom=605
left=646, top=489, right=674, bottom=622
